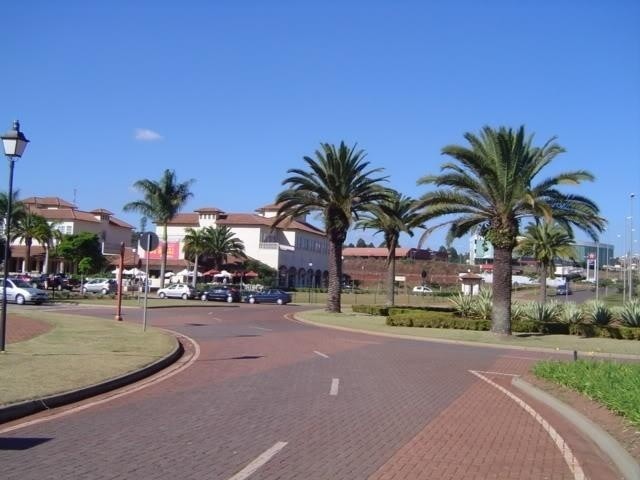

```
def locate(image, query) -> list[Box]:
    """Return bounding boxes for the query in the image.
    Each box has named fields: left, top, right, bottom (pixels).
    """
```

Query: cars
left=556, top=285, right=571, bottom=296
left=156, top=283, right=197, bottom=301
left=413, top=286, right=433, bottom=293
left=244, top=287, right=293, bottom=305
left=199, top=286, right=241, bottom=303
left=80, top=278, right=118, bottom=295
left=0, top=277, right=48, bottom=305
left=15, top=273, right=75, bottom=292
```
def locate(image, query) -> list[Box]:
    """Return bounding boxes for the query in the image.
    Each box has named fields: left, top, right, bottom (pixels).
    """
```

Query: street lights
left=131, top=249, right=136, bottom=298
left=593, top=192, right=638, bottom=304
left=1, top=119, right=31, bottom=351
left=307, top=259, right=314, bottom=302
